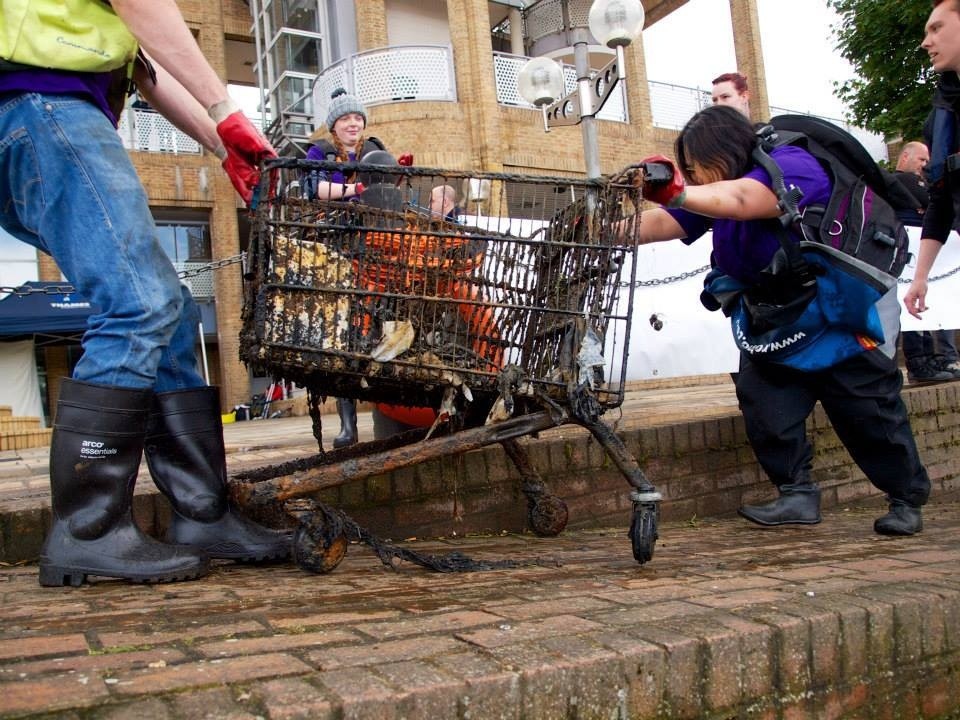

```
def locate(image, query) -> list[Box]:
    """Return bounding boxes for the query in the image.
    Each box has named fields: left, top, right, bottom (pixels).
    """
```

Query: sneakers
left=906, top=366, right=954, bottom=383
left=939, top=359, right=960, bottom=381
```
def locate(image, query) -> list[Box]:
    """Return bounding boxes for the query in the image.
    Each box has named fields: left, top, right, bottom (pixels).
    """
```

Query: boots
left=38, top=375, right=209, bottom=588
left=730, top=371, right=823, bottom=525
left=816, top=348, right=933, bottom=533
left=141, top=384, right=300, bottom=563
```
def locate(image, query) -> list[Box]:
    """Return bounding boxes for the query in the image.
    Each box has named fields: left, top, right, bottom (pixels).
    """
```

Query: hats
left=325, top=87, right=367, bottom=133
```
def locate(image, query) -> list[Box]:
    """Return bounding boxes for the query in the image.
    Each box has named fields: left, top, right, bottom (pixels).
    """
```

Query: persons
left=894, top=0, right=960, bottom=385
left=612, top=104, right=931, bottom=537
left=709, top=74, right=751, bottom=118
left=427, top=184, right=463, bottom=224
left=0, top=0, right=292, bottom=587
left=301, top=88, right=386, bottom=449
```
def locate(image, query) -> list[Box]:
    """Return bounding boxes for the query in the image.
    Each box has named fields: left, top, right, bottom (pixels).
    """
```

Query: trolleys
left=236, top=160, right=673, bottom=577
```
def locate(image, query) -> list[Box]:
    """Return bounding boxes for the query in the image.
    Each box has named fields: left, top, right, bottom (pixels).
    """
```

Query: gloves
left=640, top=154, right=688, bottom=209
left=217, top=110, right=279, bottom=209
